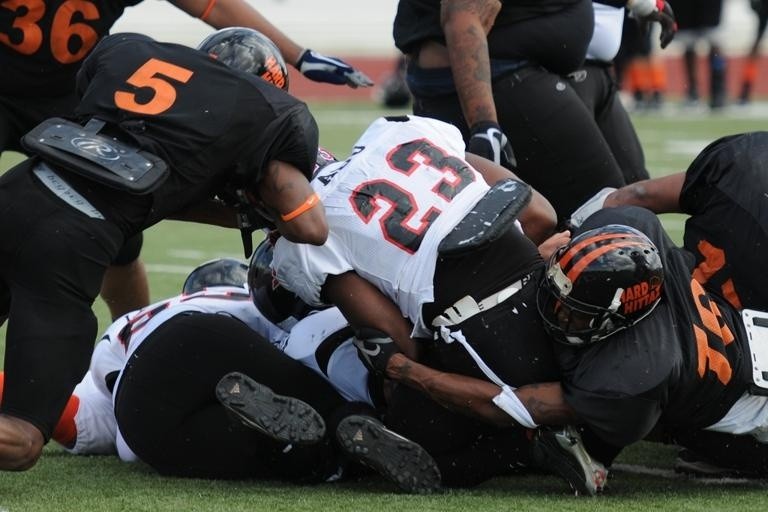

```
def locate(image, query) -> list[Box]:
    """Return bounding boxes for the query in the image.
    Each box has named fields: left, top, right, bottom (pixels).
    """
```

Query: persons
left=0, top=0, right=767, bottom=495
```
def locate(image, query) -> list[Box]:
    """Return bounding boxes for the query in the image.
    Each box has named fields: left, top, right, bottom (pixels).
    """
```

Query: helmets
left=533, top=223, right=665, bottom=348
left=183, top=258, right=251, bottom=291
left=195, top=26, right=290, bottom=91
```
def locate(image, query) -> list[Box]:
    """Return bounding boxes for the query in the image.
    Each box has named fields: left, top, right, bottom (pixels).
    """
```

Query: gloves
left=351, top=327, right=404, bottom=381
left=630, top=0, right=678, bottom=50
left=295, top=48, right=375, bottom=90
left=465, top=119, right=518, bottom=172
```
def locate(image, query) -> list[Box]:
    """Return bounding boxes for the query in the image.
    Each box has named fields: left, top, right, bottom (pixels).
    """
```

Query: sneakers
left=539, top=422, right=614, bottom=497
left=334, top=413, right=442, bottom=496
left=215, top=370, right=327, bottom=448
left=674, top=446, right=768, bottom=489
